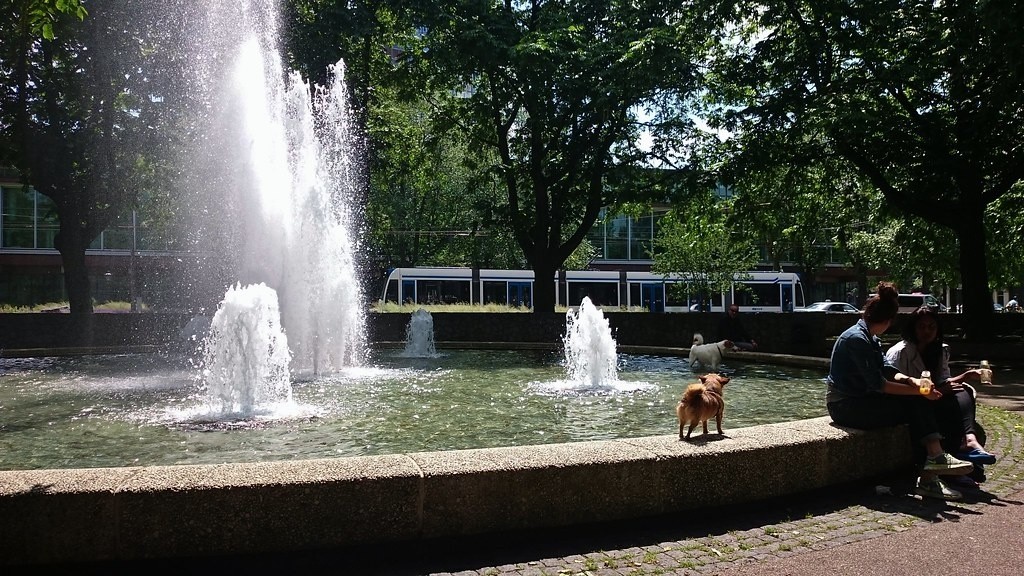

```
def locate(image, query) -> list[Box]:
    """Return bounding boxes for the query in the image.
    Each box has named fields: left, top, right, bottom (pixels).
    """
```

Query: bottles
left=980, top=361, right=992, bottom=386
left=919, top=371, right=931, bottom=395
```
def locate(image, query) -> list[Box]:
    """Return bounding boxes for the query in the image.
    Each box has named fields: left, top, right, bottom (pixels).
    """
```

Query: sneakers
left=923, top=454, right=973, bottom=475
left=915, top=475, right=962, bottom=499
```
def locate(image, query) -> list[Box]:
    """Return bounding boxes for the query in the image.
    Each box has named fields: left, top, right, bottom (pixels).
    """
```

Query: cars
left=793, top=299, right=862, bottom=313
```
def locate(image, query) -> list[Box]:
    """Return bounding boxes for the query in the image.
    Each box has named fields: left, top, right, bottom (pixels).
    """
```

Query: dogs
left=689, top=333, right=738, bottom=371
left=676, top=373, right=731, bottom=438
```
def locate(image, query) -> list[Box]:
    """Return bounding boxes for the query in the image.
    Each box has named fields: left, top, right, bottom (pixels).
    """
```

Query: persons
left=881, top=304, right=996, bottom=489
left=848, top=286, right=858, bottom=306
left=1005, top=296, right=1019, bottom=313
left=826, top=281, right=974, bottom=511
left=715, top=304, right=759, bottom=353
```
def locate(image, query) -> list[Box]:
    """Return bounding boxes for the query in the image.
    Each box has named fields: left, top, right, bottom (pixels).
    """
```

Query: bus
left=376, top=267, right=806, bottom=312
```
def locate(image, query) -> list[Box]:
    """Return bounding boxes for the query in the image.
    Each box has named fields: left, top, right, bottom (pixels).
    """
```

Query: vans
left=869, top=292, right=948, bottom=313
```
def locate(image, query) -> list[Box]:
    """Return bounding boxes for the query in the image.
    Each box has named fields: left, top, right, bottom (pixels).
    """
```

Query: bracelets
left=905, top=377, right=910, bottom=384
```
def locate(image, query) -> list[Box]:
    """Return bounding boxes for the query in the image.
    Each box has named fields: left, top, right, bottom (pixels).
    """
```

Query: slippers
left=941, top=474, right=980, bottom=489
left=954, top=446, right=996, bottom=465
